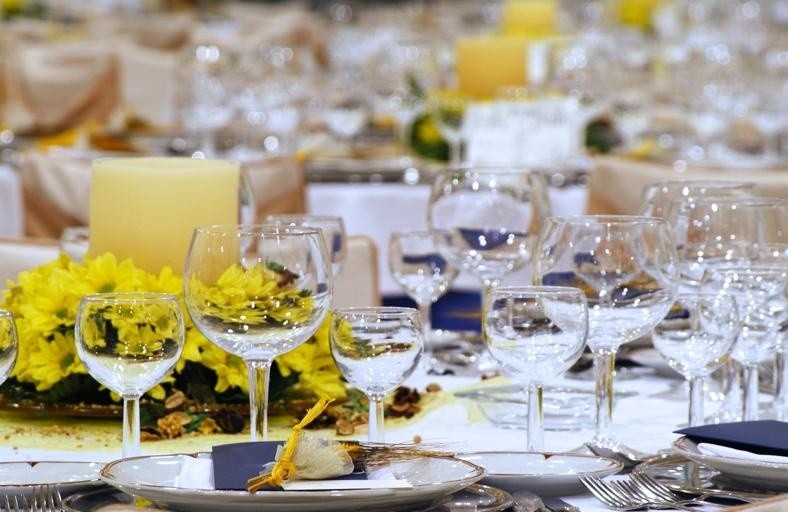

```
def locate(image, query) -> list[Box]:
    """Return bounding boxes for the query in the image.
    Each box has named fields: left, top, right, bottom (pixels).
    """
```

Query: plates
left=669, top=432, right=780, bottom=490
left=4, top=456, right=112, bottom=491
left=455, top=449, right=629, bottom=494
left=99, top=447, right=519, bottom=512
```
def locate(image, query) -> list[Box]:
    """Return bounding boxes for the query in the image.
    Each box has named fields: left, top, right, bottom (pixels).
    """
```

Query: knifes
left=531, top=484, right=584, bottom=510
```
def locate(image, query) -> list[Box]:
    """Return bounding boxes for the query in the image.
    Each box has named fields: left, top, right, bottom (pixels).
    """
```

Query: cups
left=260, top=213, right=345, bottom=278
left=653, top=292, right=739, bottom=432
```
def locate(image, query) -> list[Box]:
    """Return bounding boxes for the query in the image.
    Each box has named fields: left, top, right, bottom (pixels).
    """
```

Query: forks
left=572, top=467, right=783, bottom=512
left=6, top=477, right=122, bottom=508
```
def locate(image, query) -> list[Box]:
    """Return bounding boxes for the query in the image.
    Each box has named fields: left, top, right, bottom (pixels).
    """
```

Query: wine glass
left=540, top=213, right=683, bottom=460
left=184, top=226, right=332, bottom=441
left=1, top=310, right=17, bottom=393
left=74, top=296, right=181, bottom=458
left=328, top=309, right=427, bottom=441
left=643, top=179, right=783, bottom=494
left=385, top=229, right=464, bottom=377
left=426, top=167, right=554, bottom=377
left=482, top=287, right=593, bottom=460
